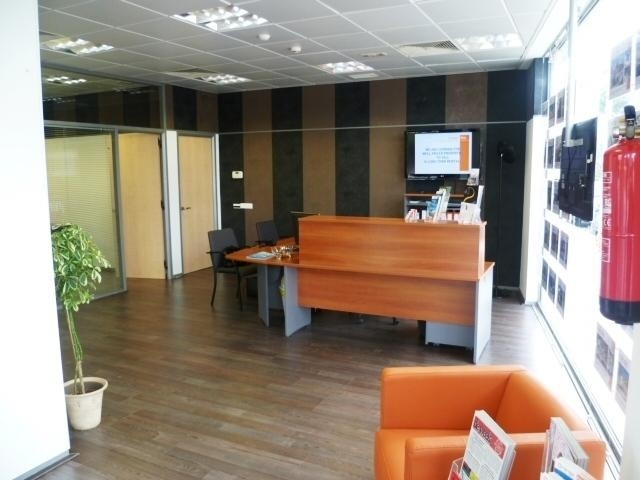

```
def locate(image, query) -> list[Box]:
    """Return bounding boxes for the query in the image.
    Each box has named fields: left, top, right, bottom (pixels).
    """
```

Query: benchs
left=225, top=220, right=495, bottom=364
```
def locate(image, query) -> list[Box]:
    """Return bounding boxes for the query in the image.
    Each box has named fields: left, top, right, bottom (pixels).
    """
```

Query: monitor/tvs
left=403, top=127, right=480, bottom=182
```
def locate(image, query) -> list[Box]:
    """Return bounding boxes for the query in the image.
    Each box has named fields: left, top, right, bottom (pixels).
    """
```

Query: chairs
left=372, top=363, right=607, bottom=480
left=206, top=222, right=279, bottom=308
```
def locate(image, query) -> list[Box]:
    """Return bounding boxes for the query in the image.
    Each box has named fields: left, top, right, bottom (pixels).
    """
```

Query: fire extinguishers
left=599, top=105, right=640, bottom=325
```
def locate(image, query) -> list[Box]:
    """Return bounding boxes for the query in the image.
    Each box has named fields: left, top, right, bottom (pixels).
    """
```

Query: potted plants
left=50, top=222, right=114, bottom=432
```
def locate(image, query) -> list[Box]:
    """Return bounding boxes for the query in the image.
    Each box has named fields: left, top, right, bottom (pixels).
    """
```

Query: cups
left=272, top=245, right=294, bottom=259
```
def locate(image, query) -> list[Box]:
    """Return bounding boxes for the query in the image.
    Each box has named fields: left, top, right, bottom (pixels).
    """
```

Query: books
left=539, top=416, right=598, bottom=479
left=456, top=410, right=515, bottom=480
left=426, top=187, right=449, bottom=220
left=246, top=251, right=276, bottom=261
left=459, top=169, right=484, bottom=224
